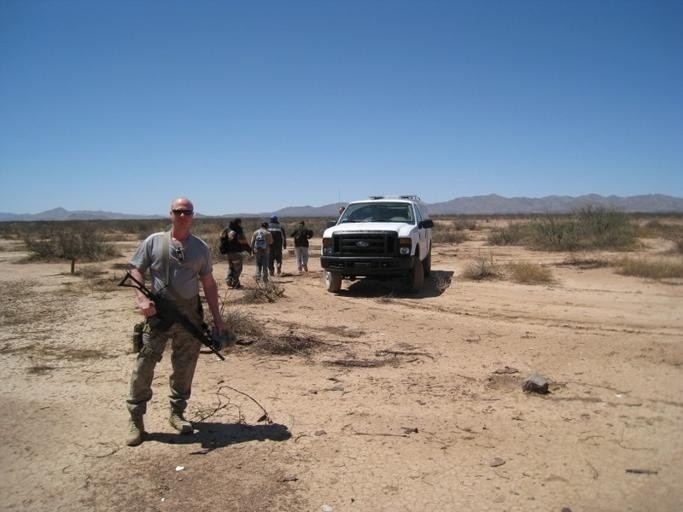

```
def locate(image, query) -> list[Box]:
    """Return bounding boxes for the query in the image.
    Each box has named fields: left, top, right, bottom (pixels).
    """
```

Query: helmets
left=270, top=215, right=277, bottom=223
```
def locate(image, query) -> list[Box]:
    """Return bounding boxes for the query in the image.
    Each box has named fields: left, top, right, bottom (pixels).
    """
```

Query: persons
left=336, top=206, right=354, bottom=222
left=266, top=215, right=287, bottom=277
left=225, top=218, right=255, bottom=288
left=123, top=196, right=225, bottom=447
left=289, top=220, right=312, bottom=275
left=250, top=221, right=273, bottom=283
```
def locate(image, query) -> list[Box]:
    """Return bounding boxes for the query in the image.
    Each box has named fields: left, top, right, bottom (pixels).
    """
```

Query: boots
left=124, top=414, right=144, bottom=446
left=169, top=407, right=193, bottom=432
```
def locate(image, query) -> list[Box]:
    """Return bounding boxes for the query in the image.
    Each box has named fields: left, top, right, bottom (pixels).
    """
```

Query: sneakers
left=277, top=266, right=280, bottom=273
left=225, top=279, right=231, bottom=287
left=302, top=264, right=308, bottom=272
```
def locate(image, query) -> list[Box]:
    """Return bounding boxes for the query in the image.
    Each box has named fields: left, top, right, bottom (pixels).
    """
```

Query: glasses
left=171, top=208, right=193, bottom=216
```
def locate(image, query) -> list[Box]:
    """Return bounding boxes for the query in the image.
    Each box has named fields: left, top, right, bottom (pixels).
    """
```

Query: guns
left=117, top=272, right=224, bottom=361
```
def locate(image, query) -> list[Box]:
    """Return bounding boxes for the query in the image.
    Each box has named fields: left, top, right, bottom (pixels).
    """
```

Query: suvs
left=319, top=195, right=432, bottom=294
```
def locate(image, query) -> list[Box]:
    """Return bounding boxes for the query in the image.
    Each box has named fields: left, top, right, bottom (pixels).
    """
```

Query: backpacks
left=217, top=227, right=231, bottom=254
left=254, top=230, right=267, bottom=249
